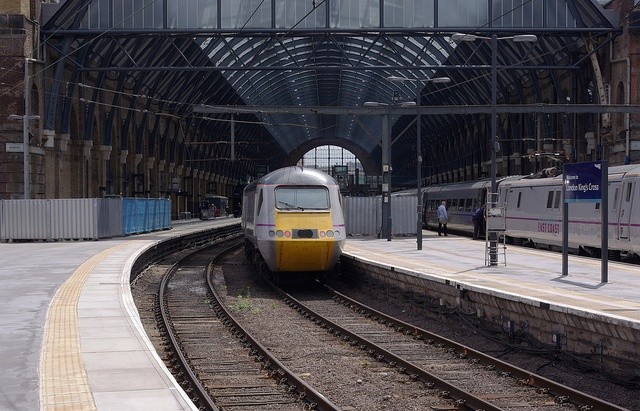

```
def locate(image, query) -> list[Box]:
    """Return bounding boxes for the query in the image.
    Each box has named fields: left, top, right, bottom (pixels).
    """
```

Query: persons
left=472, top=202, right=485, bottom=241
left=437, top=201, right=448, bottom=237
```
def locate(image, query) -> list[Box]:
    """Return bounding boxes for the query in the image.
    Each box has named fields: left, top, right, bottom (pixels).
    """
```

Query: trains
left=242, top=165, right=347, bottom=288
left=375, top=163, right=639, bottom=276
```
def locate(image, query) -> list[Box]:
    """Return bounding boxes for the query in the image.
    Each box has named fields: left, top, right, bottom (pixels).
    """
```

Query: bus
left=205, top=196, right=229, bottom=217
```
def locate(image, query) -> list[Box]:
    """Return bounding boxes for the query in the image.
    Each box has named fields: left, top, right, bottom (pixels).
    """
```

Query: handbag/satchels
left=439, top=217, right=446, bottom=224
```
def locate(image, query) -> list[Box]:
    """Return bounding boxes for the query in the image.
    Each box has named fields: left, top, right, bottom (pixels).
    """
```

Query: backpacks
left=472, top=213, right=480, bottom=223
